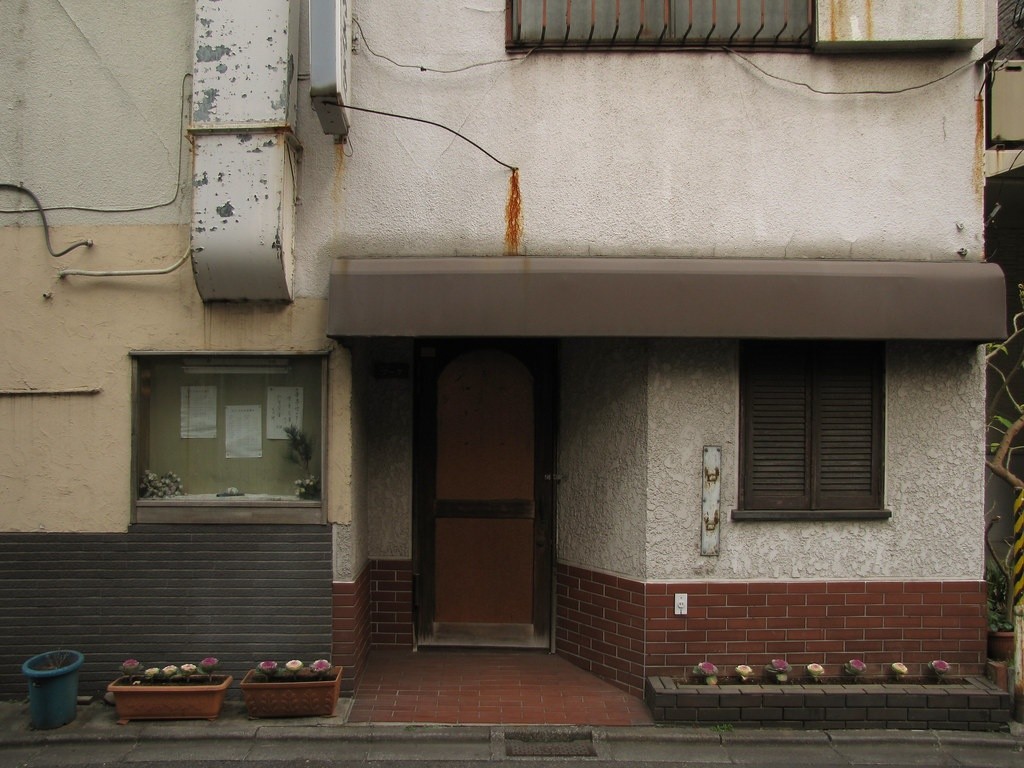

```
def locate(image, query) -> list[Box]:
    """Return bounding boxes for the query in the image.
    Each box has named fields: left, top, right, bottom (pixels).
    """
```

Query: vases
left=107, top=674, right=233, bottom=721
left=240, top=666, right=343, bottom=719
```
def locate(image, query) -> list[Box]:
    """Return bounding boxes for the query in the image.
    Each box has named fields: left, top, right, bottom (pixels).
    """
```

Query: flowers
left=119, top=656, right=219, bottom=685
left=255, top=658, right=332, bottom=680
left=690, top=660, right=951, bottom=685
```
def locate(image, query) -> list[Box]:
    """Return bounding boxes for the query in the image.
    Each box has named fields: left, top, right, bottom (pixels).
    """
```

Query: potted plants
left=22, top=643, right=85, bottom=729
left=281, top=426, right=321, bottom=501
left=987, top=599, right=1016, bottom=660
